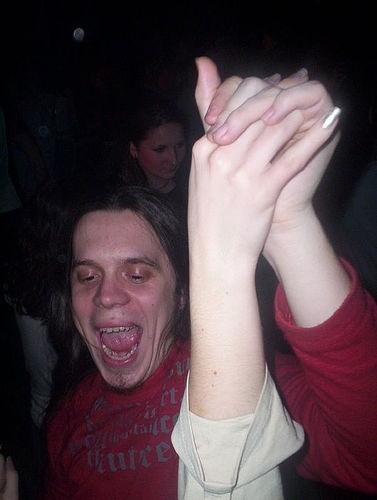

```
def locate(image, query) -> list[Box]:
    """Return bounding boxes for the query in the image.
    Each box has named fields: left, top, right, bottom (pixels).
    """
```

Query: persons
left=169, top=76, right=343, bottom=499
left=0, top=0, right=377, bottom=458
left=34, top=54, right=377, bottom=499
